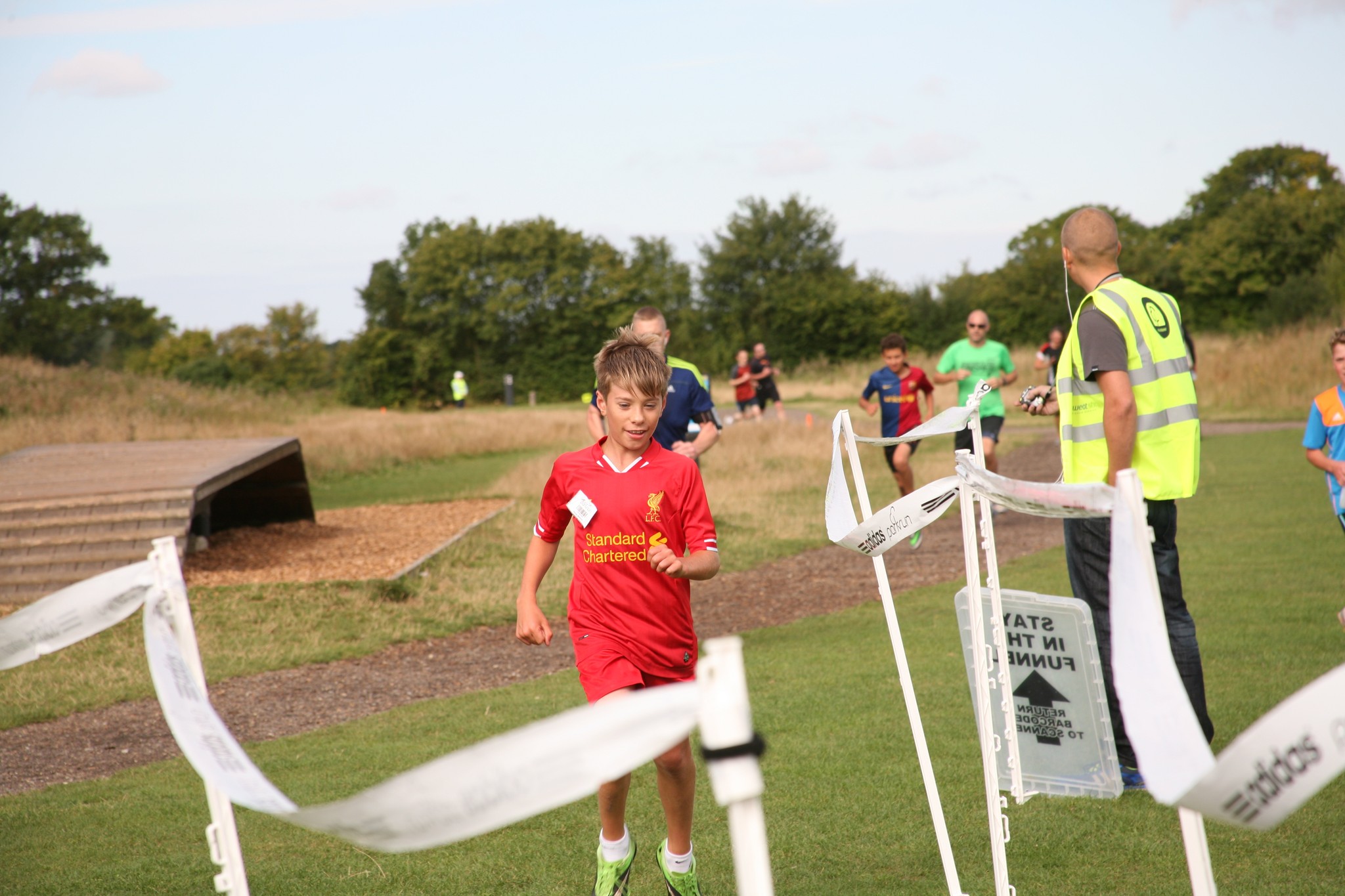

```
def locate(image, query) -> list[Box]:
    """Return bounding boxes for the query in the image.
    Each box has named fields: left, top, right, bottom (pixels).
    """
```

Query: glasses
left=967, top=322, right=987, bottom=330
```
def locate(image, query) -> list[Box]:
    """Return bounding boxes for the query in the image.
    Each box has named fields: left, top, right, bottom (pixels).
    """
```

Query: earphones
left=1064, top=260, right=1066, bottom=268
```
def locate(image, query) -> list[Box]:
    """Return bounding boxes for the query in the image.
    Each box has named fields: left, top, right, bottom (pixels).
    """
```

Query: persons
left=1303, top=329, right=1345, bottom=534
left=933, top=309, right=1015, bottom=514
left=1034, top=328, right=1066, bottom=386
left=584, top=307, right=722, bottom=461
left=858, top=334, right=935, bottom=549
left=723, top=342, right=787, bottom=425
left=450, top=370, right=469, bottom=412
left=515, top=323, right=720, bottom=896
left=1011, top=205, right=1215, bottom=789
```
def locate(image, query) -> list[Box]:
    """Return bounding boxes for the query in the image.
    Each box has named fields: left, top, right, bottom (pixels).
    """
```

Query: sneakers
left=909, top=529, right=923, bottom=549
left=656, top=837, right=702, bottom=896
left=593, top=838, right=637, bottom=896
left=1062, top=761, right=1148, bottom=791
